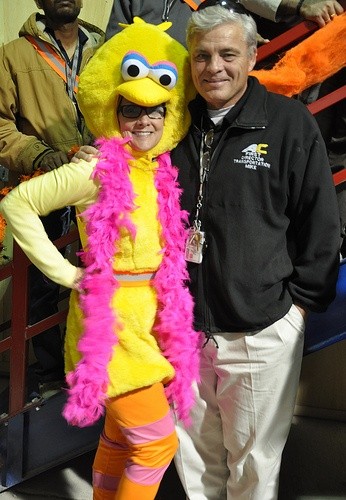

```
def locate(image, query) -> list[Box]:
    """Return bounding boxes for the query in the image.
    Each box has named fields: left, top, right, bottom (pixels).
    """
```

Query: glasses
left=199, top=127, right=213, bottom=172
left=118, top=104, right=167, bottom=120
